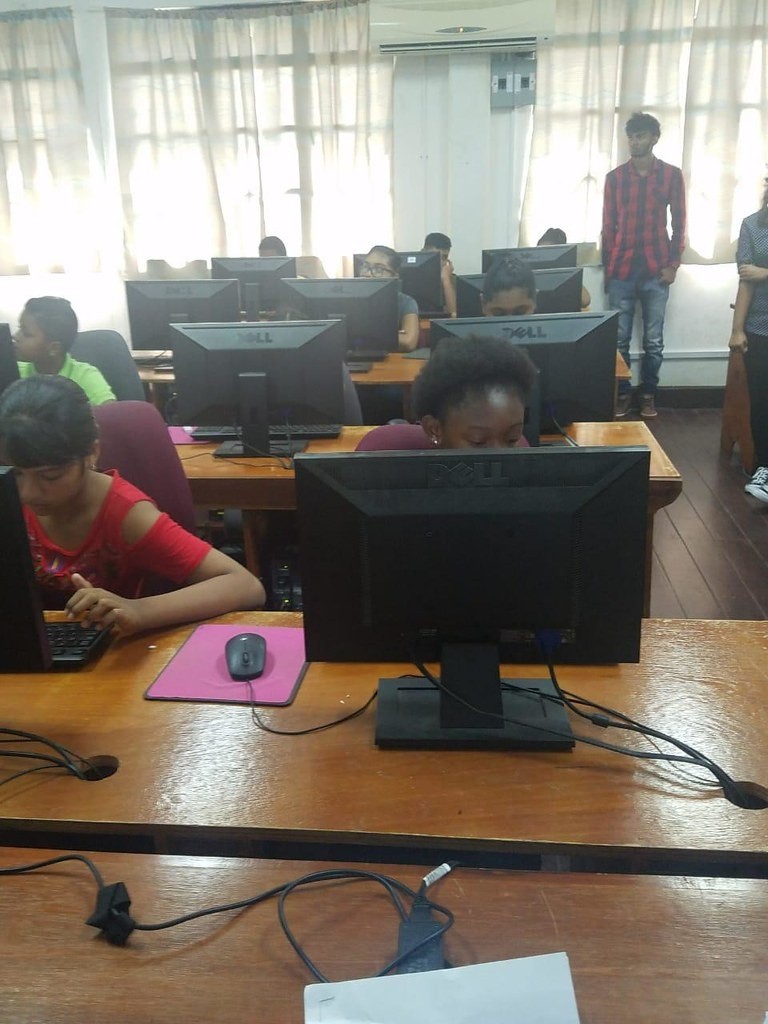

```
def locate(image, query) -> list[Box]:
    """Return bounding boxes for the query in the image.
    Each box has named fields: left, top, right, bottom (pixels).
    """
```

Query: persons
left=258, top=236, right=307, bottom=279
left=422, top=232, right=457, bottom=319
left=480, top=256, right=537, bottom=316
left=270, top=308, right=363, bottom=426
left=354, top=333, right=537, bottom=451
left=360, top=245, right=420, bottom=354
left=537, top=228, right=591, bottom=309
left=11, top=296, right=118, bottom=407
left=601, top=112, right=686, bottom=417
left=729, top=177, right=768, bottom=504
left=0, top=375, right=267, bottom=640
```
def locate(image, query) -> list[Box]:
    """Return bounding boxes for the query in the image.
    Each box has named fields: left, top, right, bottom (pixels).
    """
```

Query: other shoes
left=639, top=396, right=658, bottom=416
left=613, top=395, right=631, bottom=417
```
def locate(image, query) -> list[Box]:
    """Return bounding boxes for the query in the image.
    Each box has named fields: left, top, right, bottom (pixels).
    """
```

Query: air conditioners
left=369, top=0, right=556, bottom=56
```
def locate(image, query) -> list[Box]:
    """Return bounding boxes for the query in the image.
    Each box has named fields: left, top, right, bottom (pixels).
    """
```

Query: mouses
left=226, top=633, right=267, bottom=679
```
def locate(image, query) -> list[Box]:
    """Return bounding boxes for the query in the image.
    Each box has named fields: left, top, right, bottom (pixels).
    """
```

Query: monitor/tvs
left=126, top=243, right=651, bottom=750
left=1, top=466, right=52, bottom=674
left=0, top=323, right=21, bottom=395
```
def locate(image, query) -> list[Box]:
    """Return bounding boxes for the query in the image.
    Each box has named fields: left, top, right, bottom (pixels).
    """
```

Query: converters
left=396, top=921, right=444, bottom=975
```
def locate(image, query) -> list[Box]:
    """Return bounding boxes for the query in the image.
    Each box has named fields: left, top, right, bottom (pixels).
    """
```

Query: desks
left=0, top=610, right=768, bottom=866
left=581, top=307, right=591, bottom=311
left=719, top=304, right=756, bottom=475
left=130, top=350, right=633, bottom=421
left=92, top=421, right=683, bottom=618
left=0, top=845, right=768, bottom=1024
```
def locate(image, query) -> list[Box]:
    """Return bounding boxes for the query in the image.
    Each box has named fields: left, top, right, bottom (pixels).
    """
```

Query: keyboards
left=132, top=356, right=174, bottom=365
left=419, top=312, right=451, bottom=319
left=190, top=424, right=342, bottom=440
left=44, top=620, right=113, bottom=667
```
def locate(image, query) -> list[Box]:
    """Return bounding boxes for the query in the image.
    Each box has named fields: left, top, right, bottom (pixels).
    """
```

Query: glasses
left=358, top=262, right=394, bottom=277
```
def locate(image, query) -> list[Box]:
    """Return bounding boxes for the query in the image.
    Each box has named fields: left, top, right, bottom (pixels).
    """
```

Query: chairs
left=296, top=256, right=328, bottom=279
left=340, top=363, right=363, bottom=427
left=66, top=330, right=145, bottom=401
left=83, top=400, right=195, bottom=600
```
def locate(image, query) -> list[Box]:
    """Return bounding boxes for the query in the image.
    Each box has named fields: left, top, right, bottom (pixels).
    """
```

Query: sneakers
left=744, top=466, right=768, bottom=502
